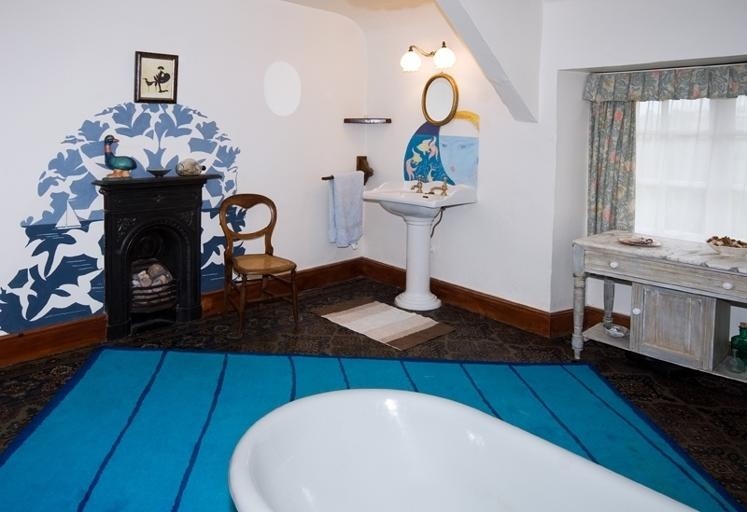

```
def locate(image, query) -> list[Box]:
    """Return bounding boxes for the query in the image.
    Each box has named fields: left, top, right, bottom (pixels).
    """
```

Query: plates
left=147, top=167, right=170, bottom=178
left=710, top=242, right=747, bottom=257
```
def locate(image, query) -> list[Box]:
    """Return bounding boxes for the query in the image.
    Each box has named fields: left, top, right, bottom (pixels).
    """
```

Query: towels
left=326, top=170, right=366, bottom=250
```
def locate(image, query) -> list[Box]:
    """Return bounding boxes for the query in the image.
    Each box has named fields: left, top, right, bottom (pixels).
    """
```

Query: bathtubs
left=225, top=388, right=701, bottom=511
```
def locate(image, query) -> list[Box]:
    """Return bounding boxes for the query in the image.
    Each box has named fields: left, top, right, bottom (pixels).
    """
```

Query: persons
left=439, top=110, right=479, bottom=186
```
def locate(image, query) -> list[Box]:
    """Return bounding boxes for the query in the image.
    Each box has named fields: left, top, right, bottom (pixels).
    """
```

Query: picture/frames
left=134, top=49, right=178, bottom=106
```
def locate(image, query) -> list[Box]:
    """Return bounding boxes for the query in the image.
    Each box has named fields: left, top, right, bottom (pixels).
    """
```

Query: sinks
left=362, top=179, right=477, bottom=216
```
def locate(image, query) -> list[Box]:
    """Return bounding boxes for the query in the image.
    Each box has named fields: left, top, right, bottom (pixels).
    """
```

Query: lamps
left=399, top=40, right=457, bottom=73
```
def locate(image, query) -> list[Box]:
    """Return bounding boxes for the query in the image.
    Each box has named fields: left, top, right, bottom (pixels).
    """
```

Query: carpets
left=313, top=296, right=457, bottom=352
left=0, top=344, right=747, bottom=512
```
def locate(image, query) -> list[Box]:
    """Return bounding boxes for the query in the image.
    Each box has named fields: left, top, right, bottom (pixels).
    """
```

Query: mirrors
left=422, top=74, right=459, bottom=126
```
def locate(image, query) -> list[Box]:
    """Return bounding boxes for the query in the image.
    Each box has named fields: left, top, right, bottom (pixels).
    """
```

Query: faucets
left=410, top=176, right=422, bottom=193
left=430, top=178, right=448, bottom=196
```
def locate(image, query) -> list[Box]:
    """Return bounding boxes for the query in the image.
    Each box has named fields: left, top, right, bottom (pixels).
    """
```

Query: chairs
left=217, top=193, right=301, bottom=338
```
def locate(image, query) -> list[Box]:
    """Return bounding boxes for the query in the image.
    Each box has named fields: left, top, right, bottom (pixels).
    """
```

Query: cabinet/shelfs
left=569, top=227, right=747, bottom=385
